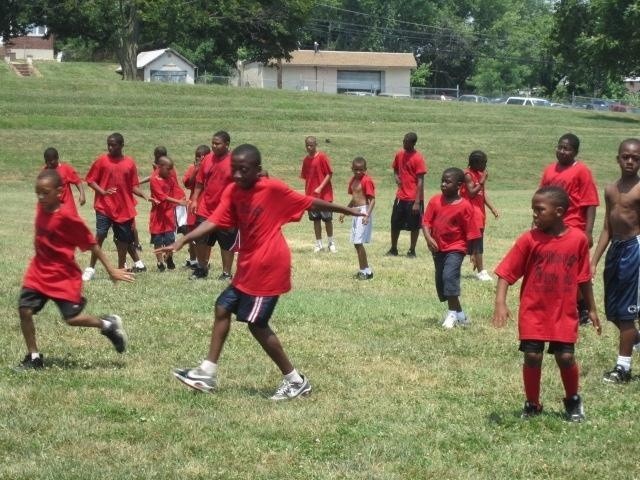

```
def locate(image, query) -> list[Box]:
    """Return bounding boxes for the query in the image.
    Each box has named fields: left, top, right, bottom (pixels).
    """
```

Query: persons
left=16, top=169, right=128, bottom=370
left=339, top=156, right=375, bottom=281
left=43, top=147, right=86, bottom=215
left=113, top=198, right=147, bottom=272
left=491, top=185, right=602, bottom=423
left=421, top=167, right=482, bottom=329
left=458, top=150, right=499, bottom=282
left=136, top=146, right=178, bottom=247
left=149, top=156, right=189, bottom=273
left=301, top=136, right=336, bottom=253
left=590, top=138, right=640, bottom=383
left=188, top=131, right=239, bottom=281
left=152, top=143, right=369, bottom=402
left=383, top=131, right=427, bottom=258
left=531, top=133, right=600, bottom=326
left=183, top=144, right=211, bottom=270
left=82, top=132, right=147, bottom=282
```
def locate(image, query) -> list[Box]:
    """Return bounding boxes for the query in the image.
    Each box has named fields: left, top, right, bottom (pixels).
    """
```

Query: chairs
left=173, top=368, right=214, bottom=393
left=128, top=261, right=232, bottom=282
left=270, top=374, right=312, bottom=403
left=14, top=353, right=44, bottom=371
left=521, top=401, right=542, bottom=417
left=102, top=316, right=127, bottom=353
left=314, top=240, right=415, bottom=279
left=443, top=310, right=468, bottom=328
left=83, top=267, right=96, bottom=282
left=576, top=301, right=590, bottom=324
left=603, top=364, right=631, bottom=384
left=478, top=269, right=491, bottom=280
left=563, top=393, right=585, bottom=425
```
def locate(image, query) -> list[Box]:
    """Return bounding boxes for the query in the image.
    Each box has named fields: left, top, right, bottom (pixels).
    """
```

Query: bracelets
left=479, top=182, right=484, bottom=186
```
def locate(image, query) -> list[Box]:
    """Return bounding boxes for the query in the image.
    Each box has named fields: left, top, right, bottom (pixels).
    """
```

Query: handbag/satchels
left=479, top=182, right=484, bottom=186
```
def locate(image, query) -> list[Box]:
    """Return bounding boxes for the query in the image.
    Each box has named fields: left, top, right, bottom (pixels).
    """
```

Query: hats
left=344, top=85, right=639, bottom=115
